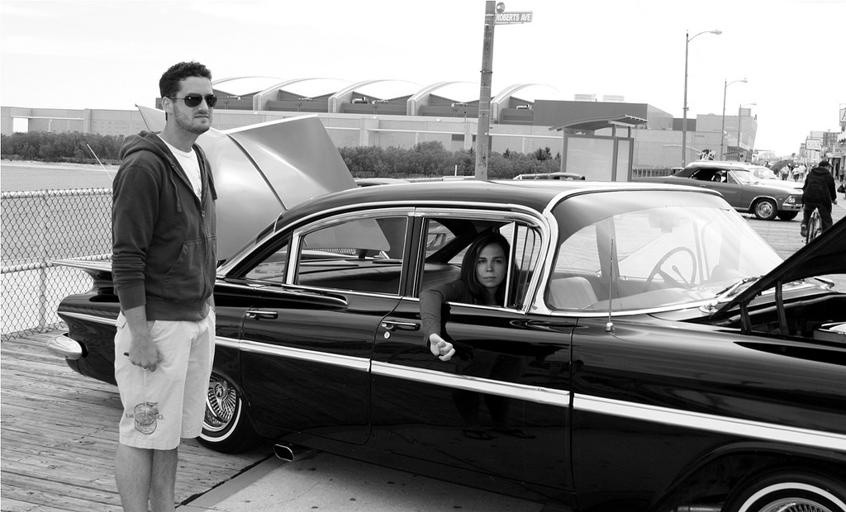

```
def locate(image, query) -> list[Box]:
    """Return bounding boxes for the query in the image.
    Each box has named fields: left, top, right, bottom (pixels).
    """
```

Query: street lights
left=720, top=80, right=748, bottom=161
left=682, top=30, right=722, bottom=167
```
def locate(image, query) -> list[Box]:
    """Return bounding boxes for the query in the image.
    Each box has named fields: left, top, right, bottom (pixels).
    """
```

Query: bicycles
left=801, top=201, right=837, bottom=245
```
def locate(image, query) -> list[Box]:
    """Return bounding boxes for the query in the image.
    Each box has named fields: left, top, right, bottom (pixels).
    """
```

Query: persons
left=764, top=160, right=807, bottom=182
left=801, top=161, right=837, bottom=237
left=419, top=233, right=522, bottom=362
left=110, top=62, right=217, bottom=512
left=713, top=174, right=721, bottom=181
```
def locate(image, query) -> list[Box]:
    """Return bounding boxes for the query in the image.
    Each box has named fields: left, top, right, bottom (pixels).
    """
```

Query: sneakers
left=799, top=224, right=807, bottom=237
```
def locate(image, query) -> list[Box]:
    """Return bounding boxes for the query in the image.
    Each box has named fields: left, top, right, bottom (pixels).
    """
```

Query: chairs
left=544, top=277, right=596, bottom=310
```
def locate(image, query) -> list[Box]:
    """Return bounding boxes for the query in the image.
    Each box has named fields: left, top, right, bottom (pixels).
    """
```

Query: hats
left=818, top=160, right=834, bottom=167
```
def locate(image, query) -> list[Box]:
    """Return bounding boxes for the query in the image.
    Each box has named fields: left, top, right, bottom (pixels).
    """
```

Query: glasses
left=169, top=93, right=217, bottom=108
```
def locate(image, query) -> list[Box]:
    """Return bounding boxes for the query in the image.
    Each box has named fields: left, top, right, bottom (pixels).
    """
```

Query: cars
left=46, top=103, right=846, bottom=512
left=632, top=161, right=804, bottom=220
